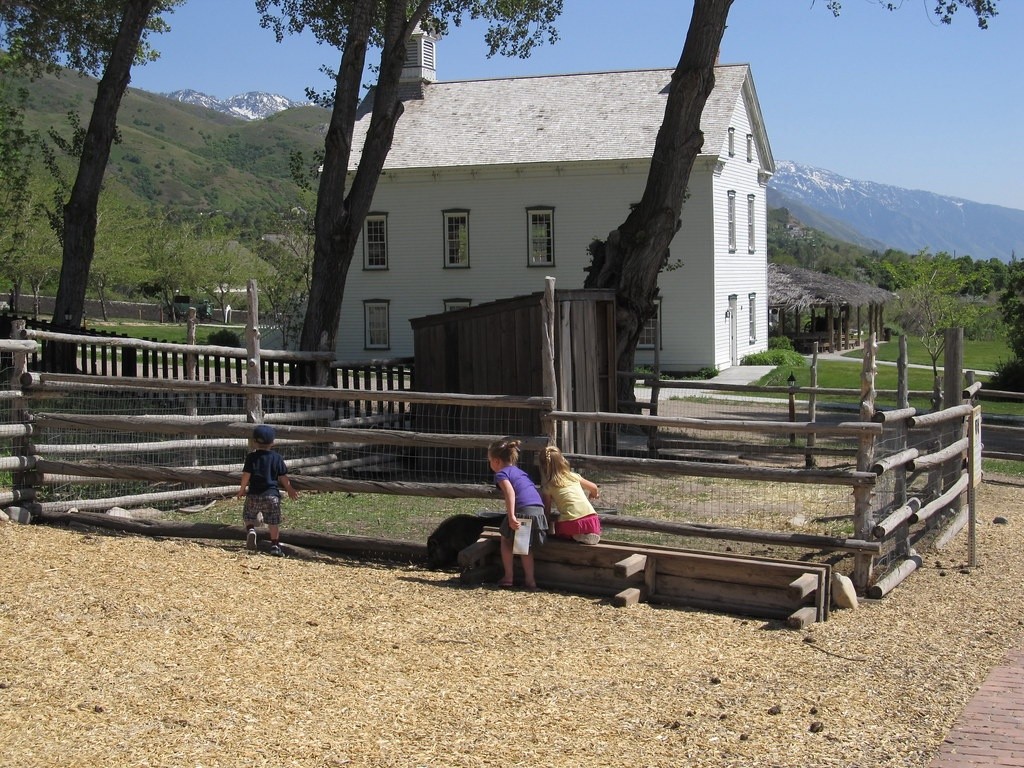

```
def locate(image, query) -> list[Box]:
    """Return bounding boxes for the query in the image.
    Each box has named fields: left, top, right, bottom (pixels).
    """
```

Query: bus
left=173, top=295, right=214, bottom=322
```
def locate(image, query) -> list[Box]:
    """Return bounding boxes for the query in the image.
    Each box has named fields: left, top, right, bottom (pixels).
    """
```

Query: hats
left=252, top=425, right=275, bottom=445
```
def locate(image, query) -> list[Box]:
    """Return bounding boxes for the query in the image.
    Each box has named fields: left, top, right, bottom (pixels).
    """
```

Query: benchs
left=457, top=525, right=832, bottom=631
left=780, top=334, right=856, bottom=352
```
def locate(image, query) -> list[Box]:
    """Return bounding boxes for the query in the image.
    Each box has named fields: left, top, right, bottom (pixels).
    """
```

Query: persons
left=489, top=438, right=548, bottom=588
left=237, top=425, right=298, bottom=556
left=540, top=446, right=601, bottom=544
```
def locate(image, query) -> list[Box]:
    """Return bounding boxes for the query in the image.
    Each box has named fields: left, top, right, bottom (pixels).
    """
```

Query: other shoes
left=490, top=580, right=512, bottom=587
left=572, top=532, right=600, bottom=544
left=514, top=580, right=537, bottom=591
left=246, top=529, right=257, bottom=551
left=271, top=545, right=283, bottom=557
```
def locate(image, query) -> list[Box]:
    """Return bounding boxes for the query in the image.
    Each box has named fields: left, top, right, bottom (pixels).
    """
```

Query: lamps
left=725, top=309, right=730, bottom=318
